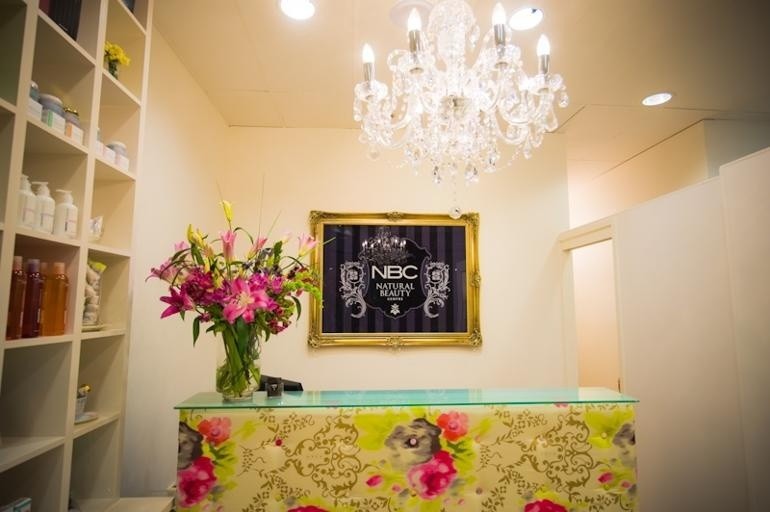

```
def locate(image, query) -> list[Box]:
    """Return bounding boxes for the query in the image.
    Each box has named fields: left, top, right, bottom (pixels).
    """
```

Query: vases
left=215, top=311, right=265, bottom=401
left=108, top=61, right=121, bottom=79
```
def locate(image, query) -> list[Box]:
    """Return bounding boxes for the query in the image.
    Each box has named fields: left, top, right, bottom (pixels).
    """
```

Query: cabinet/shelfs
left=0, top=0, right=155, bottom=512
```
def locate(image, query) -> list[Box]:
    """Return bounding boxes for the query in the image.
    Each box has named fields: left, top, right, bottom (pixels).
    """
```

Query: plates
left=82, top=324, right=107, bottom=332
left=74, top=413, right=99, bottom=425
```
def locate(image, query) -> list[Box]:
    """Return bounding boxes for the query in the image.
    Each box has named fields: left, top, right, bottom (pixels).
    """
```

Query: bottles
left=40, top=261, right=51, bottom=279
left=38, top=92, right=66, bottom=137
left=6, top=256, right=26, bottom=340
left=30, top=81, right=40, bottom=119
left=105, top=141, right=129, bottom=172
left=43, top=262, right=71, bottom=336
left=63, top=106, right=84, bottom=147
left=22, top=258, right=43, bottom=338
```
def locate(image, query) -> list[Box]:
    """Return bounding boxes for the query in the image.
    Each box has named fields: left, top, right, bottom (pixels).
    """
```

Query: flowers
left=142, top=200, right=337, bottom=394
left=103, top=41, right=134, bottom=65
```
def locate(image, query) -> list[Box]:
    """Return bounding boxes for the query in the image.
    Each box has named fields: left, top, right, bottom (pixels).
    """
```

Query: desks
left=170, top=385, right=638, bottom=512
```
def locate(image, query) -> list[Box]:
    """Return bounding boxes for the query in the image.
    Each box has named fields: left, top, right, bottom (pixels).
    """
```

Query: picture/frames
left=308, top=209, right=483, bottom=348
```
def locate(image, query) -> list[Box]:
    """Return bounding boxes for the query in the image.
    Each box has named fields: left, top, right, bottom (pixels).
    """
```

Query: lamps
left=352, top=3, right=570, bottom=186
